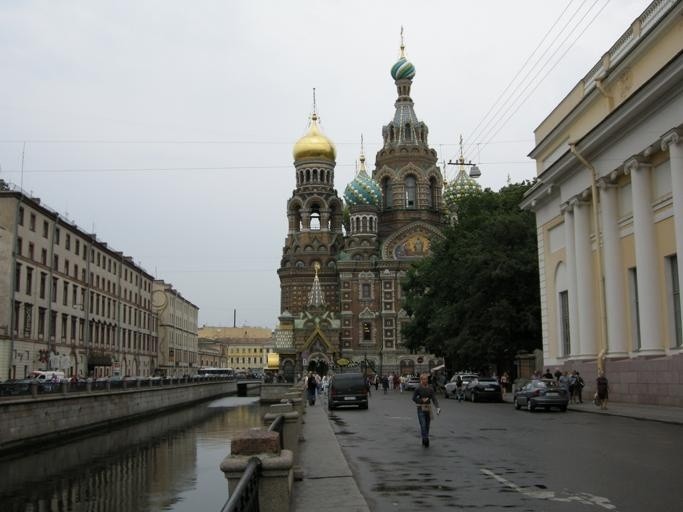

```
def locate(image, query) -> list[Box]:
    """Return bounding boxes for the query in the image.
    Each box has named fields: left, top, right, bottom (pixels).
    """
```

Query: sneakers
left=421, top=438, right=430, bottom=449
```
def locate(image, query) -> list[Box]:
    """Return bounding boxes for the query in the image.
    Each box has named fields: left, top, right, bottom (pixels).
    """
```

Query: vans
left=324, top=372, right=369, bottom=410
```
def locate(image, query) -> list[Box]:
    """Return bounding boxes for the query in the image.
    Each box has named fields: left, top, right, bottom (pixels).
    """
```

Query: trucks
left=1, top=369, right=64, bottom=395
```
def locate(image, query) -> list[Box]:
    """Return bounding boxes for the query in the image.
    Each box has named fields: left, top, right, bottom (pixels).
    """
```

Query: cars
left=512, top=378, right=570, bottom=413
left=404, top=377, right=421, bottom=391
left=463, top=377, right=503, bottom=403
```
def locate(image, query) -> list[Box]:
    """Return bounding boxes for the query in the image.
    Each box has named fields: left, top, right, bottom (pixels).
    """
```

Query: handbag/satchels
left=594, top=395, right=602, bottom=406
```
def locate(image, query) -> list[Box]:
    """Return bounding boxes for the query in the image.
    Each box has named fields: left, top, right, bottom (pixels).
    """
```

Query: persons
left=595, top=369, right=611, bottom=410
left=305, top=370, right=331, bottom=407
left=410, top=372, right=441, bottom=451
left=455, top=375, right=463, bottom=401
left=530, top=368, right=585, bottom=405
left=364, top=369, right=450, bottom=399
left=499, top=371, right=510, bottom=397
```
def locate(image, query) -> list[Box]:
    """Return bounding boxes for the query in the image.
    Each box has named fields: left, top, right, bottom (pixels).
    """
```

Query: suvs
left=442, top=373, right=479, bottom=399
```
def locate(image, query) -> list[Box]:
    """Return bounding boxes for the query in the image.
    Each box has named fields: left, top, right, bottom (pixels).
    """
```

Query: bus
left=196, top=367, right=234, bottom=381
